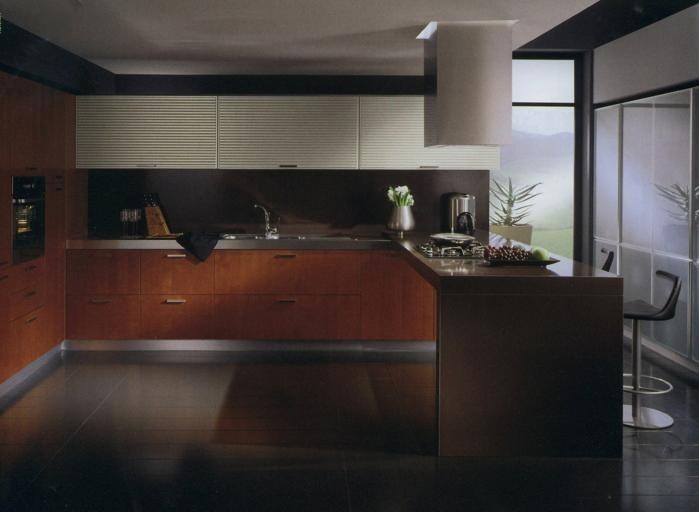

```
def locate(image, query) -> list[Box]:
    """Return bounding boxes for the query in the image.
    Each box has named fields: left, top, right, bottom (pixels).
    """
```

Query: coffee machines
left=447, top=191, right=476, bottom=235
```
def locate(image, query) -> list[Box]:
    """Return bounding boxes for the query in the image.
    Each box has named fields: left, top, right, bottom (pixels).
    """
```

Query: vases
left=387, top=205, right=416, bottom=232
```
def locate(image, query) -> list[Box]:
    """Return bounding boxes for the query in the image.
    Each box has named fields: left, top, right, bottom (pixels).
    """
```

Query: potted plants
left=653, top=181, right=699, bottom=259
left=488, top=175, right=544, bottom=246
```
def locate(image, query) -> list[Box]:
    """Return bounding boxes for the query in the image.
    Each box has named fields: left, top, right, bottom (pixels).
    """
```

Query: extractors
left=421, top=22, right=513, bottom=148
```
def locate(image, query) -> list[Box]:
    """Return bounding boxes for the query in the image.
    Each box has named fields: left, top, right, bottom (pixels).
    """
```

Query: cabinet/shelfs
left=218, top=95, right=360, bottom=170
left=0, top=70, right=65, bottom=398
left=75, top=95, right=217, bottom=170
left=66, top=240, right=395, bottom=352
left=360, top=95, right=501, bottom=171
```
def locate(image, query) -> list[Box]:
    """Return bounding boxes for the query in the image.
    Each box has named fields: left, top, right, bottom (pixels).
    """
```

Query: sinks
left=223, top=234, right=300, bottom=241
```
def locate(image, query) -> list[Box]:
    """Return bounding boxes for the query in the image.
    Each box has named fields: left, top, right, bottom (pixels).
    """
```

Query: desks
left=380, top=228, right=623, bottom=458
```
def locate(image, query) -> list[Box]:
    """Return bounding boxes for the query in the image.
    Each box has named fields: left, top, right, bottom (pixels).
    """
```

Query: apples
left=530, top=248, right=549, bottom=261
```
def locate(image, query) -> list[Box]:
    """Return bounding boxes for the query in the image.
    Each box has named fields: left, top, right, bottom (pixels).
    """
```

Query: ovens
left=10, top=174, right=44, bottom=266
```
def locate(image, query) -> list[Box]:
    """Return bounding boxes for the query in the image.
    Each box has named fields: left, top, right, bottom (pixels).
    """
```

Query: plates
left=477, top=256, right=559, bottom=267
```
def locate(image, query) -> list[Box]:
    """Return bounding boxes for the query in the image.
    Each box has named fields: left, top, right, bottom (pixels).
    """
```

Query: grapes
left=483, top=245, right=530, bottom=262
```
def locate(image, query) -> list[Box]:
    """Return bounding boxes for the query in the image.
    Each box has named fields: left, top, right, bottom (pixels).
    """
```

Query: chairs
left=600, top=248, right=614, bottom=272
left=623, top=269, right=682, bottom=429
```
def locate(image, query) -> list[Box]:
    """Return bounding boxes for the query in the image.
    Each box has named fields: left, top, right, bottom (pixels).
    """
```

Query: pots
left=428, top=231, right=477, bottom=247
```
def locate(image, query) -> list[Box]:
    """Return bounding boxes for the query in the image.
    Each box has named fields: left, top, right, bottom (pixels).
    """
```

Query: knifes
left=141, top=191, right=160, bottom=206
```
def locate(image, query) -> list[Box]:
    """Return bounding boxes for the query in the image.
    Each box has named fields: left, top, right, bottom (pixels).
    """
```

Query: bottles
left=118, top=207, right=141, bottom=240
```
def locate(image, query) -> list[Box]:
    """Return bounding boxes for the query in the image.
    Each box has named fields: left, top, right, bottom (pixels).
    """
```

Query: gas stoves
left=414, top=242, right=487, bottom=259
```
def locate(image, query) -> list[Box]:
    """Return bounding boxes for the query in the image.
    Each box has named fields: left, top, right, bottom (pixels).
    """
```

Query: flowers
left=386, top=185, right=415, bottom=208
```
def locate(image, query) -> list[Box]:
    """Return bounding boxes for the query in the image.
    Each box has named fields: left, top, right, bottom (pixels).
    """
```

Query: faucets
left=254, top=204, right=271, bottom=231
